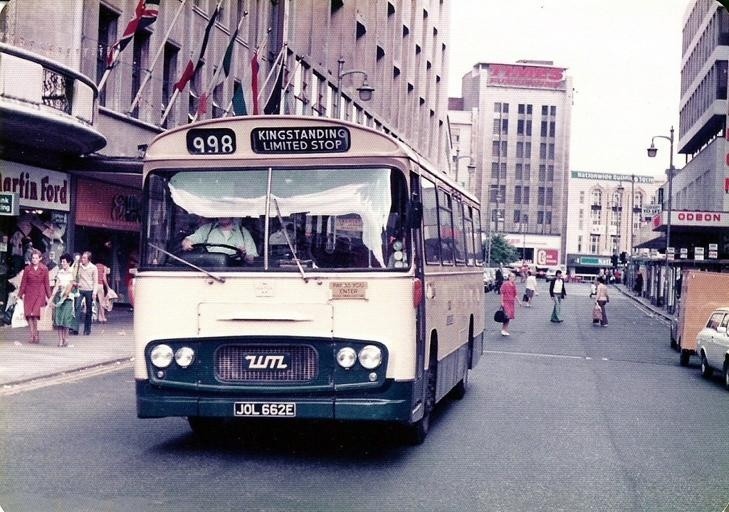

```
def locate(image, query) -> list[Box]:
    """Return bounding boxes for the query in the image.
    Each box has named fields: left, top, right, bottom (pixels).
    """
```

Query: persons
left=493, top=267, right=504, bottom=295
left=124, top=255, right=139, bottom=313
left=180, top=215, right=260, bottom=267
left=592, top=276, right=610, bottom=326
left=633, top=270, right=643, bottom=296
left=50, top=253, right=75, bottom=348
left=70, top=250, right=98, bottom=336
left=499, top=272, right=522, bottom=336
left=16, top=248, right=52, bottom=343
left=589, top=266, right=627, bottom=299
left=549, top=269, right=567, bottom=323
left=524, top=270, right=539, bottom=307
left=95, top=255, right=113, bottom=322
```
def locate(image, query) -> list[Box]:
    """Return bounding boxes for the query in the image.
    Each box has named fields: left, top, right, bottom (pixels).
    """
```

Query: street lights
left=647, top=126, right=675, bottom=315
left=453, top=146, right=476, bottom=184
left=334, top=56, right=373, bottom=121
left=616, top=174, right=634, bottom=256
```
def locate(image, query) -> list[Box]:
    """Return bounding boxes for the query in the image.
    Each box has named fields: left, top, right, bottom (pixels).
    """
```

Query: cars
left=694, top=305, right=729, bottom=387
left=483, top=261, right=599, bottom=292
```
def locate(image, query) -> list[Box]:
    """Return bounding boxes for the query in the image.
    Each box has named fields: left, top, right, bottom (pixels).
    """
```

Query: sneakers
left=29, top=336, right=38, bottom=343
left=551, top=319, right=564, bottom=322
left=593, top=323, right=608, bottom=327
left=501, top=330, right=510, bottom=336
left=58, top=339, right=69, bottom=347
left=69, top=330, right=91, bottom=335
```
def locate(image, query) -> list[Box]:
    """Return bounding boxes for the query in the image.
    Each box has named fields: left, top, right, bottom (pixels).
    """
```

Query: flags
left=101, top=1, right=307, bottom=126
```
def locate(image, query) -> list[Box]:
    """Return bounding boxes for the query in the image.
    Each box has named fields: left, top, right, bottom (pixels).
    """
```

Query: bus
left=130, top=112, right=490, bottom=453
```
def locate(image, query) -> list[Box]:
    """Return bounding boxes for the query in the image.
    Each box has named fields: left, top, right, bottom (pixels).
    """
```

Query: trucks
left=669, top=270, right=729, bottom=366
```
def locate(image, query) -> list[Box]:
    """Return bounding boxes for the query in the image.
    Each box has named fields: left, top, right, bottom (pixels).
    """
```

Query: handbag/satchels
left=494, top=306, right=506, bottom=322
left=523, top=294, right=528, bottom=301
left=592, top=303, right=602, bottom=320
